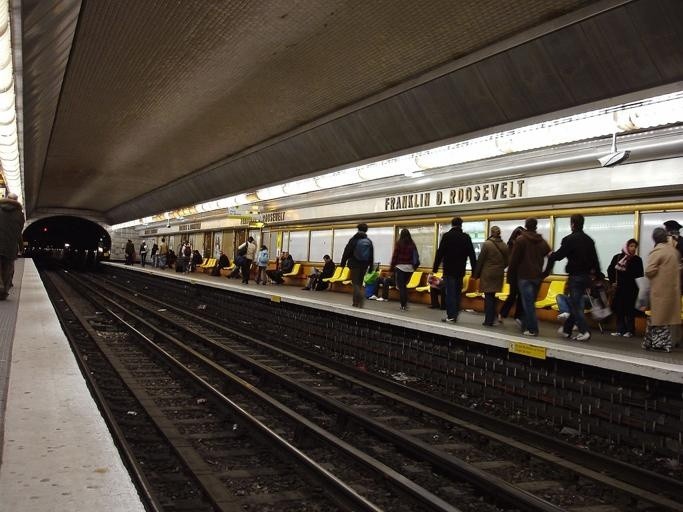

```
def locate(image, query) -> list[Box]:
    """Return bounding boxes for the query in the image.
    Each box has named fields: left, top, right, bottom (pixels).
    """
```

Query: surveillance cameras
left=166, top=224, right=170, bottom=228
left=598, top=150, right=630, bottom=166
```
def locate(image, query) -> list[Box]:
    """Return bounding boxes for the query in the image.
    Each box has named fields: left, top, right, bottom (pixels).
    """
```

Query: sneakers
left=481, top=313, right=521, bottom=327
left=610, top=329, right=635, bottom=340
left=300, top=273, right=319, bottom=290
left=573, top=332, right=592, bottom=344
left=399, top=302, right=410, bottom=312
left=426, top=302, right=447, bottom=311
left=206, top=273, right=278, bottom=285
left=557, top=327, right=571, bottom=339
left=367, top=294, right=388, bottom=303
left=556, top=312, right=571, bottom=322
left=520, top=329, right=539, bottom=338
left=440, top=316, right=458, bottom=325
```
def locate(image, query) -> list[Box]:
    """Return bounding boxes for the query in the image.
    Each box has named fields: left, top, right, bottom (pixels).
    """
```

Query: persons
left=0, top=192, right=24, bottom=300
left=9, top=231, right=25, bottom=288
left=369, top=213, right=682, bottom=356
left=339, top=223, right=374, bottom=307
left=125, top=235, right=292, bottom=289
left=301, top=255, right=335, bottom=290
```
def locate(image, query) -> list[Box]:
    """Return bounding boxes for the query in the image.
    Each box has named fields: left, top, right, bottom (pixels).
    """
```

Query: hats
left=663, top=220, right=683, bottom=231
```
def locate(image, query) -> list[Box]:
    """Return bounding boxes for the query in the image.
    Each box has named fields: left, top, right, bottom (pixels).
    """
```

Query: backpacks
left=354, top=236, right=373, bottom=261
left=184, top=245, right=192, bottom=258
left=259, top=249, right=270, bottom=264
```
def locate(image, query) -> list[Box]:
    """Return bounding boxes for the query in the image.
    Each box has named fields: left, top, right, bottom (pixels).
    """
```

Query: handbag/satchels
left=411, top=242, right=420, bottom=271
left=236, top=242, right=250, bottom=257
